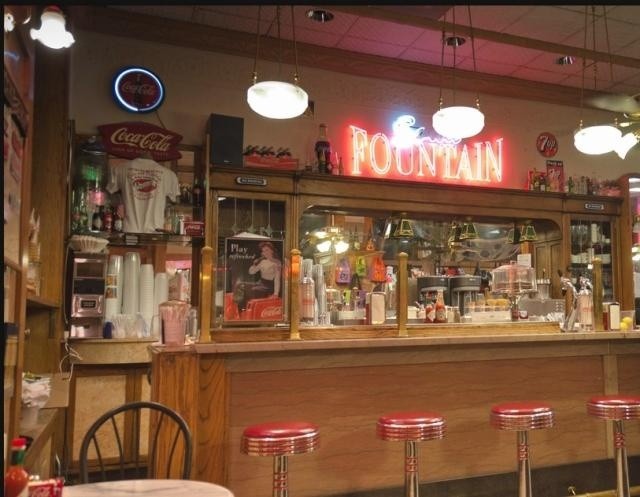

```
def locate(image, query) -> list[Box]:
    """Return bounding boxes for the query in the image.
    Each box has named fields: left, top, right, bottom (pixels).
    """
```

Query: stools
left=376, top=411, right=447, bottom=497
left=586, top=395, right=640, bottom=497
left=241, top=422, right=321, bottom=497
left=490, top=402, right=556, bottom=497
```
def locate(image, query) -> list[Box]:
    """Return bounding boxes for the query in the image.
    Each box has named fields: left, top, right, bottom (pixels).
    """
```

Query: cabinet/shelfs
left=2, top=5, right=63, bottom=479
left=64, top=132, right=210, bottom=476
left=211, top=164, right=624, bottom=328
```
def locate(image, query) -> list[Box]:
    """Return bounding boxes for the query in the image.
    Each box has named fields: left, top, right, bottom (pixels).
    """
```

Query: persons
left=239, top=241, right=281, bottom=310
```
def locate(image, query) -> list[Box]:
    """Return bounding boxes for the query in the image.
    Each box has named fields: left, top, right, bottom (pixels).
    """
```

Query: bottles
left=92, top=205, right=122, bottom=232
left=5, top=438, right=29, bottom=496
left=314, top=123, right=345, bottom=176
left=352, top=224, right=374, bottom=251
left=528, top=167, right=619, bottom=195
left=192, top=174, right=201, bottom=206
left=165, top=206, right=180, bottom=234
left=425, top=289, right=460, bottom=323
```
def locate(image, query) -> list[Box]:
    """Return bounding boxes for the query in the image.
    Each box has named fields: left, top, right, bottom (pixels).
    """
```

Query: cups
left=104, top=252, right=169, bottom=346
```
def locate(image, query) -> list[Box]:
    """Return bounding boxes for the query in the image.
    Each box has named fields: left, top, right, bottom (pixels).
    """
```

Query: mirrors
left=298, top=205, right=564, bottom=299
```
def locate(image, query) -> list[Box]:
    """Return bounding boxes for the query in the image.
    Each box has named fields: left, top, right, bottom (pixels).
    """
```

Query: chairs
left=80, top=401, right=193, bottom=483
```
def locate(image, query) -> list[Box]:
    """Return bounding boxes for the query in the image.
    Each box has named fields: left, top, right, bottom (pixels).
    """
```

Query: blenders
left=68, top=251, right=108, bottom=339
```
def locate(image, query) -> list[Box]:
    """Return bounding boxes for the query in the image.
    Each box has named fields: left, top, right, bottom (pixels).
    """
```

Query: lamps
left=573, top=5, right=622, bottom=155
left=519, top=226, right=538, bottom=243
left=247, top=5, right=309, bottom=120
left=384, top=220, right=397, bottom=239
left=432, top=5, right=484, bottom=139
left=314, top=228, right=350, bottom=253
left=31, top=5, right=76, bottom=50
left=394, top=219, right=414, bottom=240
left=460, top=222, right=477, bottom=241
left=506, top=226, right=518, bottom=244
left=449, top=224, right=463, bottom=242
left=615, top=94, right=640, bottom=160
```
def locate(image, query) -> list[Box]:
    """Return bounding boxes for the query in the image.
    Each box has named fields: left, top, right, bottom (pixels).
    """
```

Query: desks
left=20, top=479, right=234, bottom=497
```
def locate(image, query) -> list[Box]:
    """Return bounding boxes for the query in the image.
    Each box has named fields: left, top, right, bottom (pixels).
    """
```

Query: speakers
left=206, top=111, right=246, bottom=167
